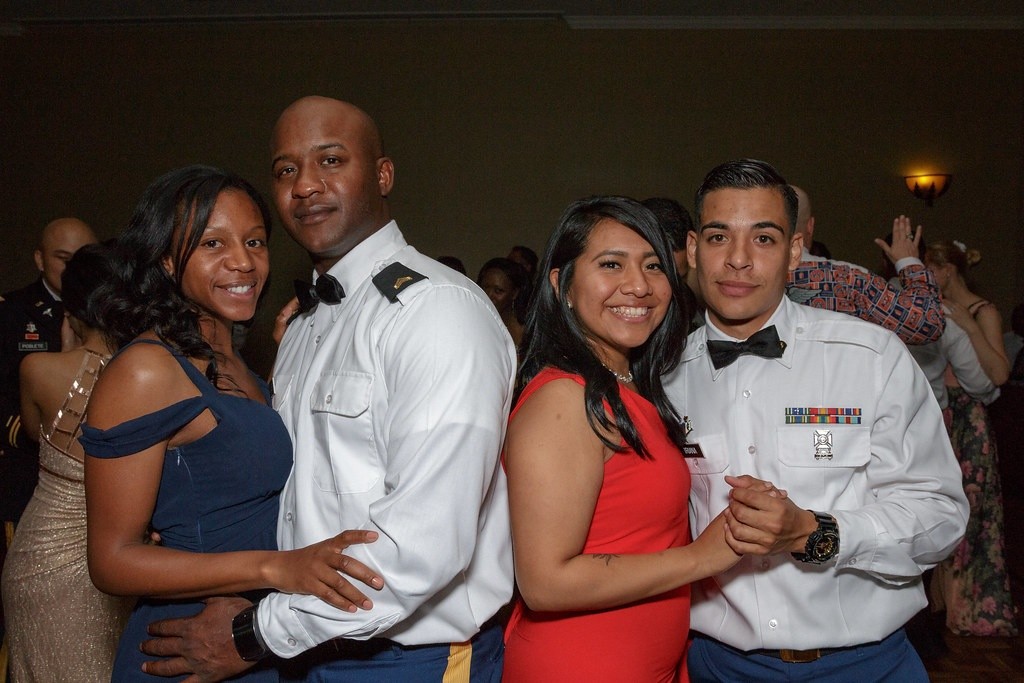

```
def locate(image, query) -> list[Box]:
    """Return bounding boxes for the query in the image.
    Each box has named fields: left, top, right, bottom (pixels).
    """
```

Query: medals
left=814, top=429, right=833, bottom=461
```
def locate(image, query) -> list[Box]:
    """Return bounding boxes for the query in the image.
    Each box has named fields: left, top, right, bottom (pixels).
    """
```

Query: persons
left=642, top=195, right=704, bottom=337
left=83, top=164, right=386, bottom=683
left=138, top=94, right=518, bottom=683
left=438, top=244, right=537, bottom=347
left=784, top=183, right=1024, bottom=665
left=500, top=193, right=788, bottom=683
left=0, top=215, right=139, bottom=683
left=652, top=156, right=971, bottom=683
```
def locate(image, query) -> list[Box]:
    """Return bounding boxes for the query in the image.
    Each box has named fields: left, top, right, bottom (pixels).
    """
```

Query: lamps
left=906, top=173, right=950, bottom=206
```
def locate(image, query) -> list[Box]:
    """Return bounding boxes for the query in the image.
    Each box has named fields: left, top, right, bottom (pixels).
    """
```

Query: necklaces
left=601, top=362, right=633, bottom=384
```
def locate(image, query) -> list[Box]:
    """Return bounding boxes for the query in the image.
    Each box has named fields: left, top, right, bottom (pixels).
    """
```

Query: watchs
left=790, top=509, right=840, bottom=566
left=230, top=603, right=288, bottom=666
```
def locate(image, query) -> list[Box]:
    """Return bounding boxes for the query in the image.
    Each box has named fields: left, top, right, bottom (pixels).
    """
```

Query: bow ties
left=295, top=274, right=346, bottom=312
left=706, top=324, right=788, bottom=369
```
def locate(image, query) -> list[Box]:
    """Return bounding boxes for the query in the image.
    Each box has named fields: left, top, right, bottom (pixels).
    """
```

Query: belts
left=760, top=646, right=842, bottom=664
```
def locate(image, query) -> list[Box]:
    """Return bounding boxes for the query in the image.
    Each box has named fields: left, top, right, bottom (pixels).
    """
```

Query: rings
left=906, top=234, right=913, bottom=239
